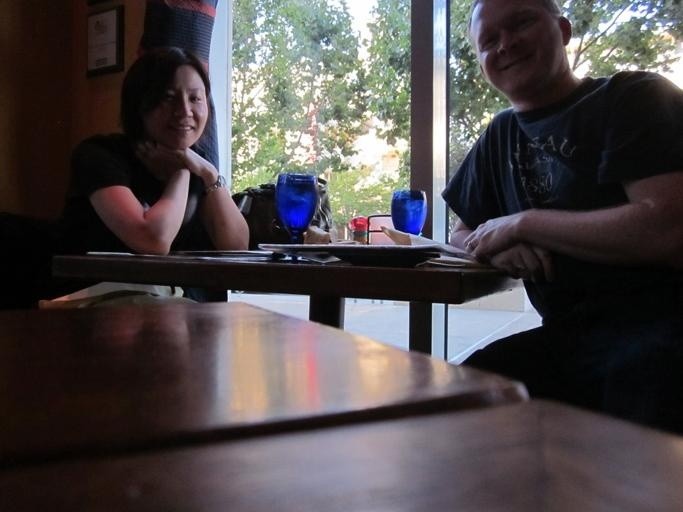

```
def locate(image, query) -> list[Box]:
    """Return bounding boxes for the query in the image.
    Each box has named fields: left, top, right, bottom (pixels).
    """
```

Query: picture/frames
left=85, top=5, right=125, bottom=78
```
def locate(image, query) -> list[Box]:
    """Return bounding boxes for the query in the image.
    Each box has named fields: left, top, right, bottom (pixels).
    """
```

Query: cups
left=390, top=189, right=427, bottom=236
left=274, top=173, right=320, bottom=263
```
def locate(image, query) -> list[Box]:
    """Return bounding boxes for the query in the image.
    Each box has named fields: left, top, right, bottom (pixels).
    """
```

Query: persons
left=446, top=0, right=683, bottom=436
left=52, top=47, right=249, bottom=255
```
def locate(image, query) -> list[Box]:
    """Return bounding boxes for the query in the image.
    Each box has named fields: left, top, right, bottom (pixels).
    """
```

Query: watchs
left=205, top=176, right=227, bottom=193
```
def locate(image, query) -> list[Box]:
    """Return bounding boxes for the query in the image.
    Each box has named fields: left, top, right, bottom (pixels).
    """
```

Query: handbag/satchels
left=230, top=177, right=334, bottom=251
